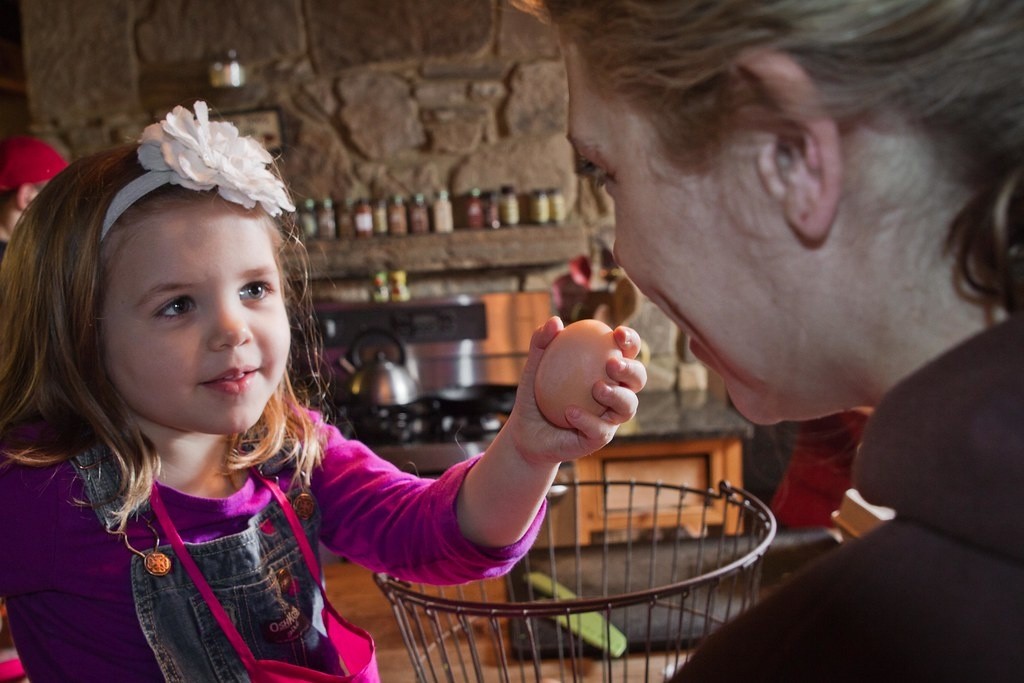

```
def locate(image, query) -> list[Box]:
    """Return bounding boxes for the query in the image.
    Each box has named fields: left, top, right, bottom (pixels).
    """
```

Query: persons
left=512, top=1, right=1024, bottom=682
left=0, top=136, right=69, bottom=266
left=0, top=100, right=647, bottom=683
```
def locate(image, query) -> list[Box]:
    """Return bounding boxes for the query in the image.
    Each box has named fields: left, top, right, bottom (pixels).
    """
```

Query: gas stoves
left=295, top=300, right=574, bottom=472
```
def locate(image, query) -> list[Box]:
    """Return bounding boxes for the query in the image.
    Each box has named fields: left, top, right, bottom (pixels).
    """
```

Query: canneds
left=293, top=184, right=567, bottom=236
left=368, top=267, right=409, bottom=303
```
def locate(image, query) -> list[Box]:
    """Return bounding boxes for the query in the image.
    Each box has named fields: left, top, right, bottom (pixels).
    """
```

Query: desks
left=311, top=527, right=844, bottom=683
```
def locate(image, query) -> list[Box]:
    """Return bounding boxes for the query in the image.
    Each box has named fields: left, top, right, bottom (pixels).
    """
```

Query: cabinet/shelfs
left=572, top=436, right=747, bottom=551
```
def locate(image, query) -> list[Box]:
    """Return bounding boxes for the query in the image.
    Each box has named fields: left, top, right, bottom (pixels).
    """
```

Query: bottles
left=467, top=188, right=483, bottom=226
left=549, top=189, right=566, bottom=225
left=375, top=199, right=388, bottom=235
left=530, top=190, right=550, bottom=225
left=390, top=197, right=407, bottom=234
left=500, top=186, right=520, bottom=224
left=355, top=199, right=373, bottom=238
left=434, top=192, right=454, bottom=232
left=339, top=199, right=355, bottom=237
left=300, top=197, right=318, bottom=237
left=486, top=191, right=499, bottom=224
left=411, top=195, right=430, bottom=231
left=319, top=200, right=336, bottom=239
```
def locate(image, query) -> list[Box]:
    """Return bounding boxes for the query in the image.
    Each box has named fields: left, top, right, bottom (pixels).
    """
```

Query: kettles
left=333, top=329, right=420, bottom=405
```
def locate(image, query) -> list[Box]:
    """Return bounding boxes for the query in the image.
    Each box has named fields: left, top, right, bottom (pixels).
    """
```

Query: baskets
left=369, top=475, right=782, bottom=683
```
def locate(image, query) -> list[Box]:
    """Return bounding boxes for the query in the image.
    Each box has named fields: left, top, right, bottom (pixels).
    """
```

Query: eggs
left=534, top=319, right=625, bottom=428
